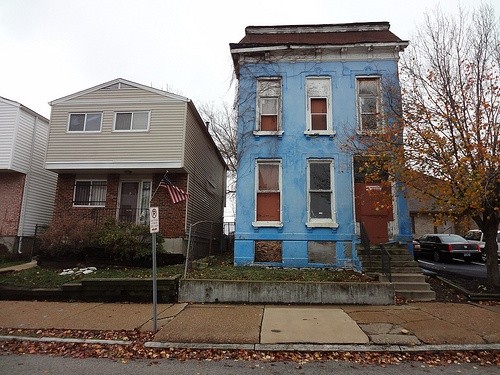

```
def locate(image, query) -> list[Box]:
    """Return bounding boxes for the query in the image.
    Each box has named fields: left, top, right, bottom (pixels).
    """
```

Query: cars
left=419, top=233, right=480, bottom=264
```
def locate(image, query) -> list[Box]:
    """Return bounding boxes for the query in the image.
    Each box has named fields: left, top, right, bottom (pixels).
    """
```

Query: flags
left=159, top=175, right=191, bottom=204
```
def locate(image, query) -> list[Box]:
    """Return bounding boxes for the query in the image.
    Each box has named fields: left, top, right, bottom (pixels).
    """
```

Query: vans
left=463, top=228, right=500, bottom=264
